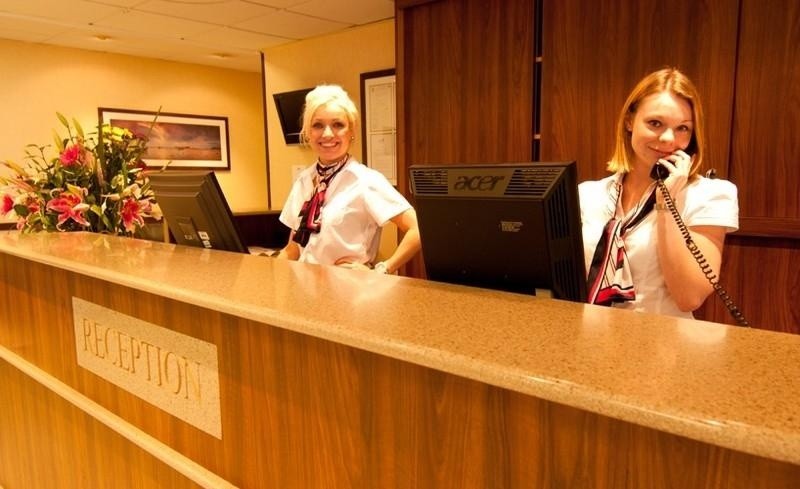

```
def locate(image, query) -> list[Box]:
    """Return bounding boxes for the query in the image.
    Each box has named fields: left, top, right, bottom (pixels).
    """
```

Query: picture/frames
left=98, top=108, right=230, bottom=170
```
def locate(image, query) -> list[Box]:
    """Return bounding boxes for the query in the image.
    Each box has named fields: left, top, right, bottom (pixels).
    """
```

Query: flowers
left=0, top=105, right=172, bottom=239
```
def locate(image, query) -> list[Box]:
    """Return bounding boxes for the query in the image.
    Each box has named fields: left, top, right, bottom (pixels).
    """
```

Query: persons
left=275, top=82, right=422, bottom=275
left=579, top=66, right=740, bottom=320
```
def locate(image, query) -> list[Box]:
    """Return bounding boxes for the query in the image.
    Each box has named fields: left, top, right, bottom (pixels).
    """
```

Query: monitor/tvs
left=273, top=88, right=317, bottom=145
left=409, top=160, right=590, bottom=303
left=146, top=171, right=250, bottom=254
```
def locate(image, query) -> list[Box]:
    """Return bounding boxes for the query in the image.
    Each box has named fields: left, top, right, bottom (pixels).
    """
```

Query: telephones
left=650, top=138, right=697, bottom=180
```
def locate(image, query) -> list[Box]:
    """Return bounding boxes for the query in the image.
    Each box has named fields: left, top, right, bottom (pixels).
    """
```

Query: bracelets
left=654, top=198, right=679, bottom=210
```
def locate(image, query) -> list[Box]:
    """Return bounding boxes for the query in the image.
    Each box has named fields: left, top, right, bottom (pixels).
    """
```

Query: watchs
left=374, top=261, right=387, bottom=274
